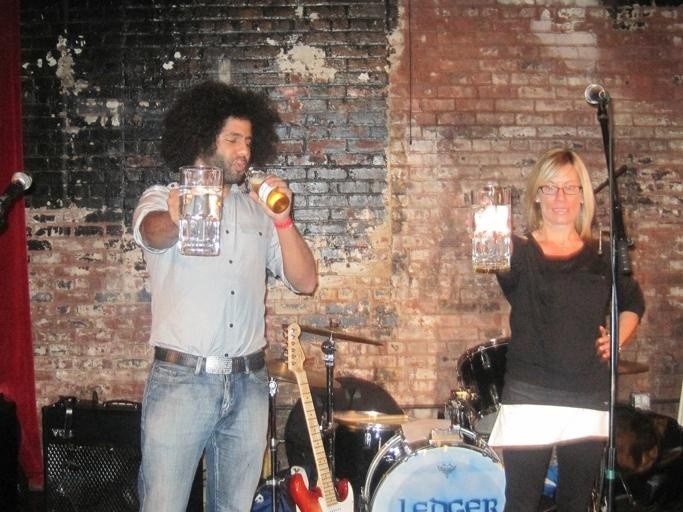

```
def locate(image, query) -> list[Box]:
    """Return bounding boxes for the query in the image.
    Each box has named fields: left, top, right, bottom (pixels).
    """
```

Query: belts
left=154, top=346, right=265, bottom=375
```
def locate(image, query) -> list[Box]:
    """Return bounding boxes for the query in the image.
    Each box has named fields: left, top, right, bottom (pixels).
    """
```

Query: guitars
left=282, top=324, right=357, bottom=511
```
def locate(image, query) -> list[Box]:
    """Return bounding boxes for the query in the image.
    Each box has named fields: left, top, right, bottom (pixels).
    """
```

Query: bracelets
left=271, top=216, right=292, bottom=229
left=607, top=333, right=623, bottom=352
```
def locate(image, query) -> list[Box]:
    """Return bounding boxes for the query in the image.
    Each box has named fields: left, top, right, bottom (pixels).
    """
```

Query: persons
left=612, top=401, right=683, bottom=512
left=463, top=148, right=646, bottom=511
left=129, top=79, right=317, bottom=512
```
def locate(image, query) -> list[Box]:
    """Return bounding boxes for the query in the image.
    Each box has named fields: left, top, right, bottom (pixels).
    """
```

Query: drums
left=360, top=422, right=505, bottom=512
left=444, top=392, right=476, bottom=429
left=456, top=335, right=511, bottom=436
left=328, top=407, right=407, bottom=511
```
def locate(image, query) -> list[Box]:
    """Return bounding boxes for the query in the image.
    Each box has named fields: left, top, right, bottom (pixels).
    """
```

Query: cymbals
left=281, top=320, right=384, bottom=349
left=266, top=359, right=342, bottom=389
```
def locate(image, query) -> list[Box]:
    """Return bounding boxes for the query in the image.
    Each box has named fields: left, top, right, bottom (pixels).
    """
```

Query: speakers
left=41, top=397, right=140, bottom=512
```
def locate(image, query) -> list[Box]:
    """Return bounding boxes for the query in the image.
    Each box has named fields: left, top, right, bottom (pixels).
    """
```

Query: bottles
left=237, top=158, right=290, bottom=212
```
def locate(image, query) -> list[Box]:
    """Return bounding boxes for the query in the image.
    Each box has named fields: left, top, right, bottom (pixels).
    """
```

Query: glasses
left=539, top=185, right=583, bottom=195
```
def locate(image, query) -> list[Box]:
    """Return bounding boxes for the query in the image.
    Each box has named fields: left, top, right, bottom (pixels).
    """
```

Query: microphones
left=0, top=171, right=33, bottom=206
left=584, top=84, right=608, bottom=106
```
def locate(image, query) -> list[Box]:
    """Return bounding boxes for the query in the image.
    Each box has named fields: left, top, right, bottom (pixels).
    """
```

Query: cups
left=178, top=164, right=222, bottom=258
left=469, top=186, right=512, bottom=272
